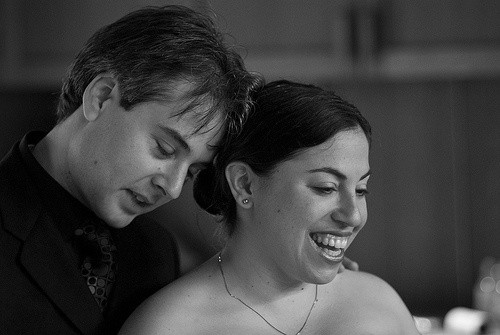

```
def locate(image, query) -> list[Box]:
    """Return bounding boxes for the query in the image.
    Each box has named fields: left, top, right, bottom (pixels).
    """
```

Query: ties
left=74, top=219, right=119, bottom=315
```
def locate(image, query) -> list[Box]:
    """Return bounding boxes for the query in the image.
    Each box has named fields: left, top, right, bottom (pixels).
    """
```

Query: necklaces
left=216, top=248, right=323, bottom=335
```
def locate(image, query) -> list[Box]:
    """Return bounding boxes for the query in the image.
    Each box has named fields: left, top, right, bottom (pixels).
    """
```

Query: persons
left=113, top=78, right=424, bottom=335
left=0, top=3, right=266, bottom=335
